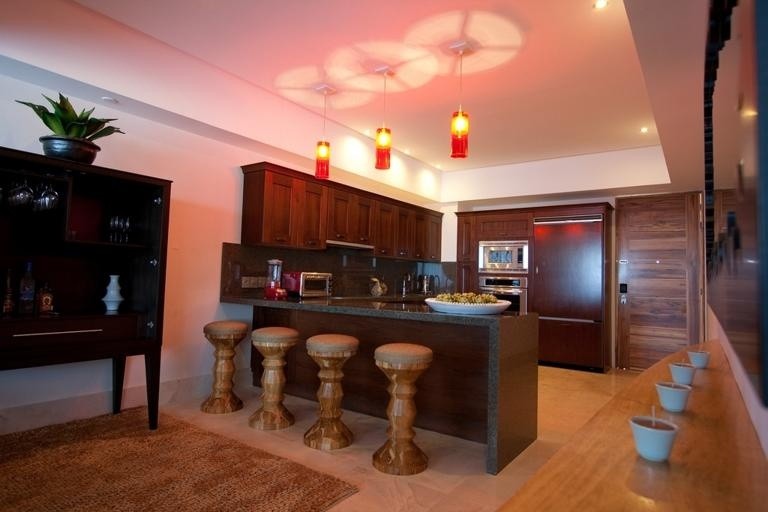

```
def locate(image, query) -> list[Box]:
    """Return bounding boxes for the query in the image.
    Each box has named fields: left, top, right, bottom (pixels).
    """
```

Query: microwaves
left=284, top=271, right=334, bottom=298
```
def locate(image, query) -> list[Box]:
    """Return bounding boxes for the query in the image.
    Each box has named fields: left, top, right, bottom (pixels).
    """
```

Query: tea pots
left=369, top=276, right=389, bottom=296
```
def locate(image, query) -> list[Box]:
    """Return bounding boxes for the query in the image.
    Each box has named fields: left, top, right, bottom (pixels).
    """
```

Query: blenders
left=263, top=260, right=288, bottom=300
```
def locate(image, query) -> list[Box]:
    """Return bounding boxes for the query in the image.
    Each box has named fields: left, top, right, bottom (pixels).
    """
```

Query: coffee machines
left=417, top=274, right=433, bottom=295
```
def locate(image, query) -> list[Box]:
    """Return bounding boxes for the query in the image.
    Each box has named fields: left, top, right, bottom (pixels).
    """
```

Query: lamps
left=312, top=82, right=334, bottom=182
left=447, top=36, right=473, bottom=160
left=372, top=60, right=393, bottom=170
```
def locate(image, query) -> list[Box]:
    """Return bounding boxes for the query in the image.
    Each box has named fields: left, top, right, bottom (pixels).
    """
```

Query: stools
left=369, top=342, right=435, bottom=478
left=195, top=320, right=249, bottom=415
left=245, top=327, right=305, bottom=432
left=301, top=332, right=363, bottom=450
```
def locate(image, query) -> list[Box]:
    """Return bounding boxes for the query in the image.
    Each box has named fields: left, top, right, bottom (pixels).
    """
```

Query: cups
left=628, top=416, right=680, bottom=463
left=686, top=350, right=711, bottom=370
left=624, top=455, right=671, bottom=501
left=654, top=380, right=691, bottom=413
left=668, top=362, right=696, bottom=385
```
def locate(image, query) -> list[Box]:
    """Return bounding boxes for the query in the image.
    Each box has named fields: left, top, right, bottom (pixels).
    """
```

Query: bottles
left=101, top=272, right=125, bottom=311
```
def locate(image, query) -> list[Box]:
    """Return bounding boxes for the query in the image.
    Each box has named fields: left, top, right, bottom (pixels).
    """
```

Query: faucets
left=401, top=272, right=411, bottom=297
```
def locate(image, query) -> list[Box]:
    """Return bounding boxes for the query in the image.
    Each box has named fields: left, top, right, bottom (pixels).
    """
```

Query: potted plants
left=13, top=89, right=131, bottom=167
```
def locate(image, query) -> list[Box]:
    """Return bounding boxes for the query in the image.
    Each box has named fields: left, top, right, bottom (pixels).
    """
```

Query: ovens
left=478, top=240, right=528, bottom=313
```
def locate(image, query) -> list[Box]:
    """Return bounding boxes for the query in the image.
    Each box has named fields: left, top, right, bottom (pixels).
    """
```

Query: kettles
left=399, top=272, right=412, bottom=297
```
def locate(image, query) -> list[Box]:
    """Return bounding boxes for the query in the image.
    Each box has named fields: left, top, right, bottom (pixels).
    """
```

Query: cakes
left=436, top=292, right=497, bottom=304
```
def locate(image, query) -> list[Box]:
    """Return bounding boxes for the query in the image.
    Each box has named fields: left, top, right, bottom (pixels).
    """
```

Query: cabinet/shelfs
left=371, top=191, right=413, bottom=263
left=239, top=160, right=324, bottom=250
left=474, top=207, right=537, bottom=242
left=0, top=143, right=176, bottom=434
left=324, top=178, right=377, bottom=250
left=411, top=201, right=444, bottom=263
left=452, top=209, right=476, bottom=291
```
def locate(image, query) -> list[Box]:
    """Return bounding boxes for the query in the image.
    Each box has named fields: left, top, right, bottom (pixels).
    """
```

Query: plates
left=425, top=298, right=511, bottom=314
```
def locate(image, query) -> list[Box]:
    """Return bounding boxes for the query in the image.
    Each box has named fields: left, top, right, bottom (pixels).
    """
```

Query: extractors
left=326, top=240, right=375, bottom=249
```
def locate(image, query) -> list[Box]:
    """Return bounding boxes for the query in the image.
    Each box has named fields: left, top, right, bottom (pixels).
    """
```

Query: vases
left=665, top=360, right=699, bottom=387
left=650, top=382, right=695, bottom=413
left=686, top=347, right=715, bottom=370
left=627, top=411, right=680, bottom=467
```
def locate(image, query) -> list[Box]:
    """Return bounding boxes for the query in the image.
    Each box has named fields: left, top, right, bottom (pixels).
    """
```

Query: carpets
left=0, top=396, right=359, bottom=511
